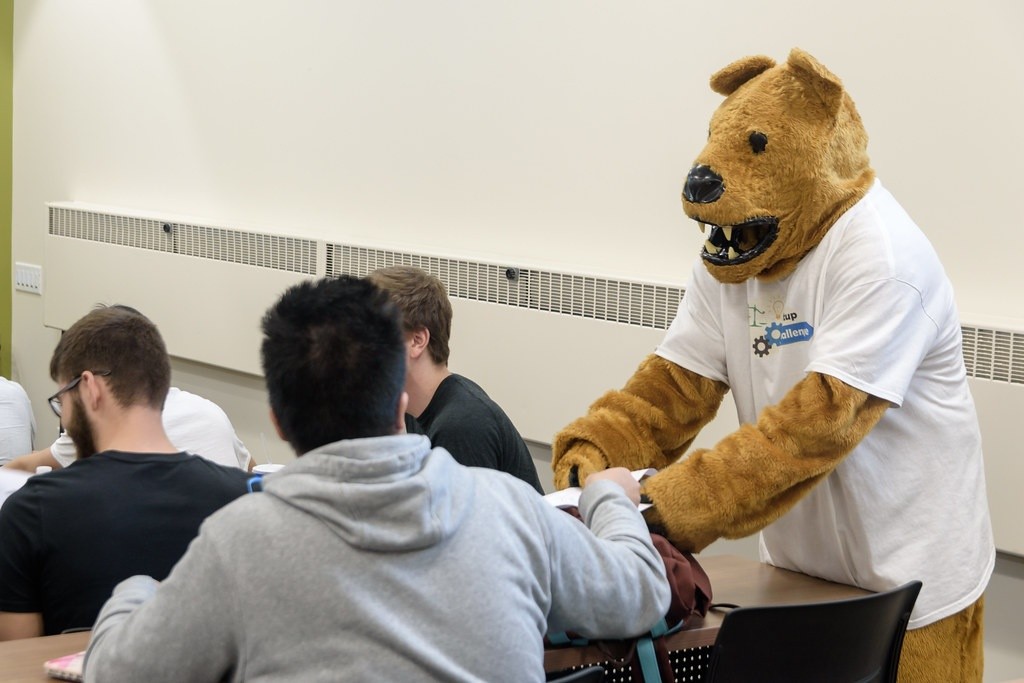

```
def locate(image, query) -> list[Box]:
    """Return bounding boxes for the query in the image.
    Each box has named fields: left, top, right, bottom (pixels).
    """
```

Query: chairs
left=701, top=580, right=921, bottom=683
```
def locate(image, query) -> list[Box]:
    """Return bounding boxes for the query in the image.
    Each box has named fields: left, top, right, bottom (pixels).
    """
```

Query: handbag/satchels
left=543, top=506, right=740, bottom=683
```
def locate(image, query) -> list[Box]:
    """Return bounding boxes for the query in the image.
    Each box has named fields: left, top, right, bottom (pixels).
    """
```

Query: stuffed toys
left=552, top=48, right=996, bottom=683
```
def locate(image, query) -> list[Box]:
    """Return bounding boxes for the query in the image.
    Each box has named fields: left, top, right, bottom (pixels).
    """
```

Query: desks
left=0, top=550, right=881, bottom=683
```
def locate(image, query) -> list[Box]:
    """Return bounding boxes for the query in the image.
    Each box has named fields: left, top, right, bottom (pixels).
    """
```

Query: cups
left=247, top=464, right=285, bottom=493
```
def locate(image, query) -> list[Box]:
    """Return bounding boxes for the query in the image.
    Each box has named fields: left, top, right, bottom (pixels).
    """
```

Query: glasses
left=48, top=370, right=111, bottom=417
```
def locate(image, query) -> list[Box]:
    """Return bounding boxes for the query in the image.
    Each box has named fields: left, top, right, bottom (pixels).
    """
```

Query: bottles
left=36, top=465, right=52, bottom=473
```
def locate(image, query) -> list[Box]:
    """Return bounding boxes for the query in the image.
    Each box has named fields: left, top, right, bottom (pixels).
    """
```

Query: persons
left=0, top=308, right=266, bottom=641
left=1, top=304, right=257, bottom=472
left=84, top=273, right=672, bottom=683
left=367, top=265, right=547, bottom=497
left=0, top=344, right=36, bottom=467
